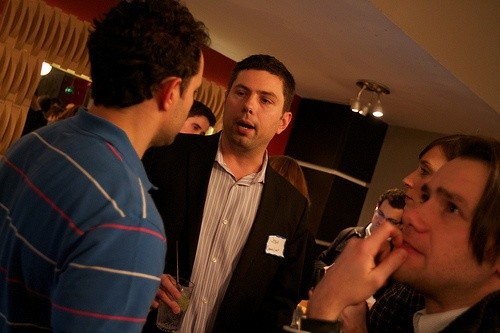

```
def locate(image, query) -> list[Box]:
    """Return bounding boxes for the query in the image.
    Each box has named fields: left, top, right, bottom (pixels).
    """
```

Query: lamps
left=351, top=80, right=391, bottom=117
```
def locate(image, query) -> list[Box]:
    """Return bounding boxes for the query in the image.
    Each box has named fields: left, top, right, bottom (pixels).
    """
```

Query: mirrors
left=22, top=62, right=92, bottom=138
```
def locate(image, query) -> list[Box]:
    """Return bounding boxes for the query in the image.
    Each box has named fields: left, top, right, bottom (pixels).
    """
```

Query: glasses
left=377, top=210, right=401, bottom=224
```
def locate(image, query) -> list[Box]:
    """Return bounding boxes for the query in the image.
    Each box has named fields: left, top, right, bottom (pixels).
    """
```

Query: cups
left=157, top=278, right=195, bottom=333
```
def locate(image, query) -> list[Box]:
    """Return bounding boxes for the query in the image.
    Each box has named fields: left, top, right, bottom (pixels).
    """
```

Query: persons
left=21, top=96, right=51, bottom=136
left=31, top=89, right=48, bottom=111
left=0, top=0, right=209, bottom=333
left=269, top=155, right=317, bottom=300
left=51, top=97, right=61, bottom=112
left=179, top=100, right=216, bottom=135
left=403, top=134, right=471, bottom=212
left=313, top=188, right=407, bottom=283
left=141, top=54, right=310, bottom=333
left=297, top=136, right=500, bottom=333
